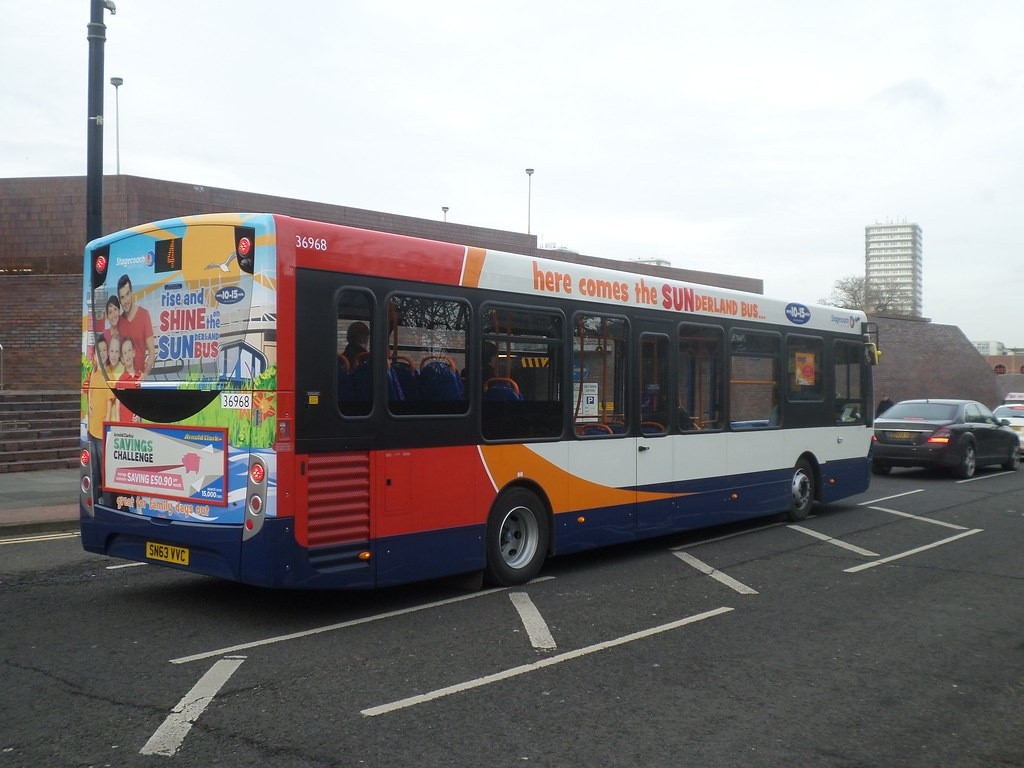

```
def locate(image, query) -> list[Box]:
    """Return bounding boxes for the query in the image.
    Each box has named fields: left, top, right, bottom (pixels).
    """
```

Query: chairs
left=576, top=416, right=701, bottom=438
left=339, top=352, right=524, bottom=410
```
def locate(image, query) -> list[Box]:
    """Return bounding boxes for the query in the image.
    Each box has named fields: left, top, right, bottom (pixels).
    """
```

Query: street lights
left=525, top=167, right=534, bottom=236
left=441, top=205, right=450, bottom=221
left=111, top=76, right=123, bottom=175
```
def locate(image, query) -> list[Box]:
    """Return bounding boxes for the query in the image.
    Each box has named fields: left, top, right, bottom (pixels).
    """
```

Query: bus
left=80, top=197, right=882, bottom=592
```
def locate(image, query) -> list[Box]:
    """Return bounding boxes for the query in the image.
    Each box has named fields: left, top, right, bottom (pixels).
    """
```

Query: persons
left=875, top=393, right=893, bottom=418
left=86, top=274, right=155, bottom=506
left=461, top=341, right=498, bottom=384
left=342, top=320, right=372, bottom=373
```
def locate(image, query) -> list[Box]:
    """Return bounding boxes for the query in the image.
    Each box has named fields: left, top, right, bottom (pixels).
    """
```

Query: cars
left=994, top=392, right=1024, bottom=460
left=873, top=399, right=1020, bottom=479
